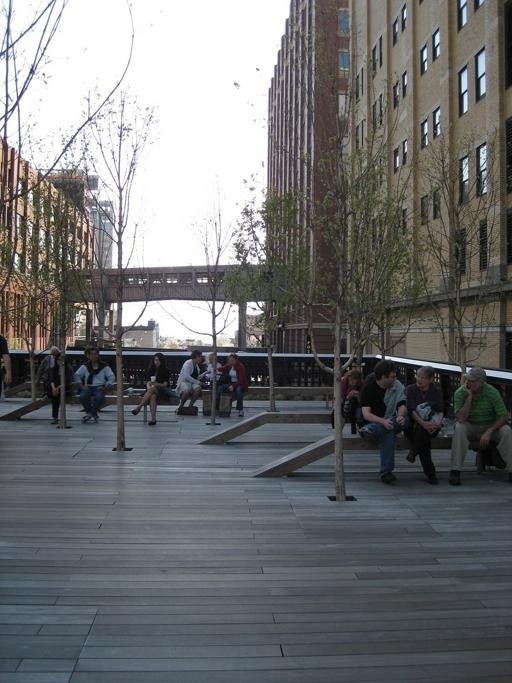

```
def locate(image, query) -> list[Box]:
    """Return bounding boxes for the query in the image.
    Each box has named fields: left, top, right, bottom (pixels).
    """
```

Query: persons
left=175, top=350, right=211, bottom=415
left=76, top=347, right=92, bottom=394
left=46, top=352, right=74, bottom=424
left=331, top=369, right=367, bottom=431
left=402, top=363, right=445, bottom=484
left=215, top=352, right=249, bottom=417
left=132, top=351, right=171, bottom=426
left=358, top=358, right=407, bottom=484
left=34, top=345, right=60, bottom=397
left=74, top=345, right=116, bottom=423
left=448, top=365, right=512, bottom=486
left=203, top=352, right=222, bottom=379
left=0, top=333, right=13, bottom=400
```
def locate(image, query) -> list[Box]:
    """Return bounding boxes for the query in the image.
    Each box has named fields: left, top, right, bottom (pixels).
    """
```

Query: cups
left=150, top=376, right=156, bottom=388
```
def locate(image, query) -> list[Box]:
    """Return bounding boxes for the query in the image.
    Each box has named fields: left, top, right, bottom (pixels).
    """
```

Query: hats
left=460, top=366, right=488, bottom=382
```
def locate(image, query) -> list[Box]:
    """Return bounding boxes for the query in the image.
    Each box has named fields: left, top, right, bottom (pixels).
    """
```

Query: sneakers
left=405, top=448, right=419, bottom=462
left=425, top=470, right=441, bottom=485
left=238, top=409, right=245, bottom=416
left=80, top=413, right=101, bottom=424
left=448, top=466, right=462, bottom=486
left=382, top=470, right=398, bottom=484
left=130, top=407, right=140, bottom=415
left=147, top=419, right=157, bottom=425
left=358, top=425, right=380, bottom=444
